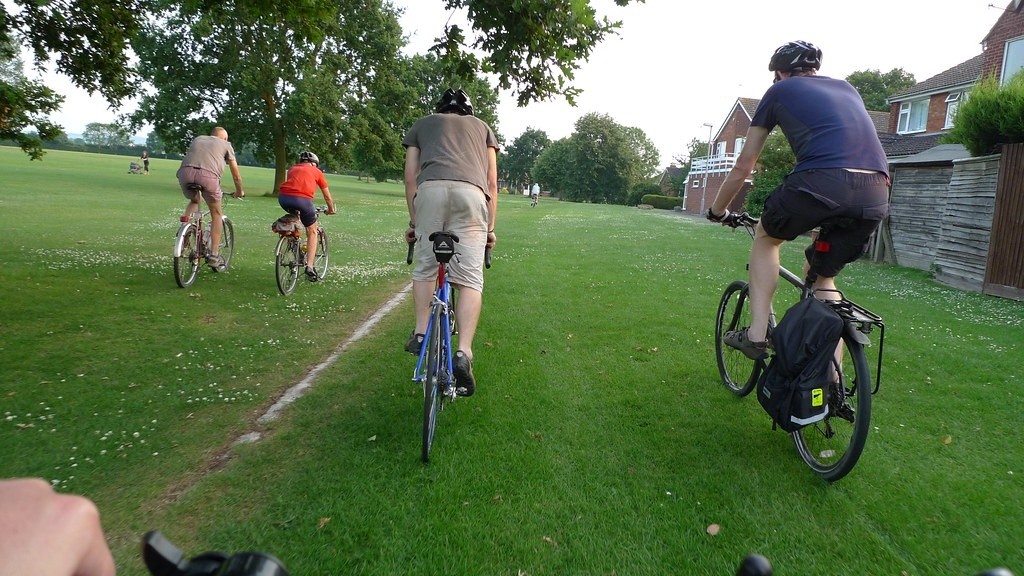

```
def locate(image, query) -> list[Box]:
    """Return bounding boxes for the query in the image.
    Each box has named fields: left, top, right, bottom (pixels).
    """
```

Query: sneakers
left=176, top=226, right=191, bottom=237
left=208, top=255, right=224, bottom=267
left=452, top=350, right=476, bottom=397
left=404, top=329, right=428, bottom=353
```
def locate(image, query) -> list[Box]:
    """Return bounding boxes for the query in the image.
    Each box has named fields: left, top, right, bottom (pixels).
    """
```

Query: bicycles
left=532, top=194, right=537, bottom=207
left=406, top=229, right=492, bottom=462
left=715, top=211, right=886, bottom=482
left=274, top=202, right=338, bottom=296
left=173, top=183, right=245, bottom=288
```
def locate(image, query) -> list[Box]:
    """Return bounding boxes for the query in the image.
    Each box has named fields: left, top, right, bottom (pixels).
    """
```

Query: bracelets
left=489, top=229, right=494, bottom=233
left=709, top=208, right=726, bottom=220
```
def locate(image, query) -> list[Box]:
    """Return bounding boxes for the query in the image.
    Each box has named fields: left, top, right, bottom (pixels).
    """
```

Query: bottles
left=302, top=238, right=307, bottom=252
left=202, top=221, right=211, bottom=242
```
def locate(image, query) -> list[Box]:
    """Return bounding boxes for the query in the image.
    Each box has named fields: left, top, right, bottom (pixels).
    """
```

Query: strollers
left=127, top=159, right=143, bottom=174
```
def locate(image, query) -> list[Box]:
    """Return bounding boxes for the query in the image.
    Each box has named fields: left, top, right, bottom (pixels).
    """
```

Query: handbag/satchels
left=757, top=298, right=843, bottom=433
left=272, top=213, right=302, bottom=232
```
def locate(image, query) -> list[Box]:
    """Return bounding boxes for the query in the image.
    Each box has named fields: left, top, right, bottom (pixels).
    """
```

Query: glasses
left=773, top=78, right=777, bottom=84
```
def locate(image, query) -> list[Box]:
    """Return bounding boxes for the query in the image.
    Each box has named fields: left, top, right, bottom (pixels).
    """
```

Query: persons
left=531, top=183, right=540, bottom=206
left=705, top=40, right=890, bottom=400
left=139, top=151, right=150, bottom=175
left=176, top=127, right=244, bottom=268
left=401, top=87, right=500, bottom=397
left=278, top=151, right=336, bottom=282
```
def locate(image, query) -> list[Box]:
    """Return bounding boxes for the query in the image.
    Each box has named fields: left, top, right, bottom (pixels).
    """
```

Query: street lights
left=699, top=124, right=713, bottom=216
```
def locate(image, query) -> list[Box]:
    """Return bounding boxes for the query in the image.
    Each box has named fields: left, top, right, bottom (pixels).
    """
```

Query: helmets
left=436, top=87, right=474, bottom=116
left=769, top=40, right=823, bottom=71
left=300, top=152, right=319, bottom=167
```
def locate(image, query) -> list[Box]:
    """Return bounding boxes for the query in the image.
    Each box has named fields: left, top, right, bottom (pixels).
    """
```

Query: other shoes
left=304, top=266, right=317, bottom=277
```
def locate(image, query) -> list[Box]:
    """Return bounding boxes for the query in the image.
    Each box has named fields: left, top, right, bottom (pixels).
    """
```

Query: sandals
left=723, top=326, right=769, bottom=360
left=830, top=383, right=841, bottom=398
